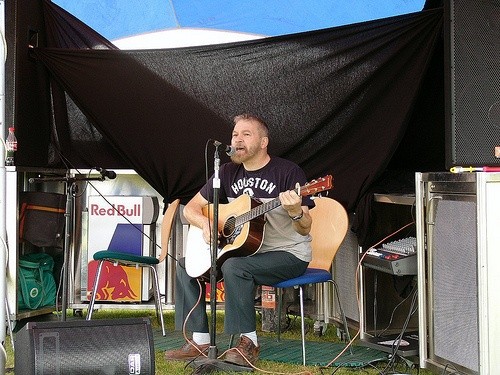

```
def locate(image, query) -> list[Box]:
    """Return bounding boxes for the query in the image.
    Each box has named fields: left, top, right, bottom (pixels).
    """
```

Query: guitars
left=185, top=174, right=336, bottom=286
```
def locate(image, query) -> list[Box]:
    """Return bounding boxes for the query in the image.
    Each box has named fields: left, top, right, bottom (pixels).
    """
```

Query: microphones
left=96, top=167, right=116, bottom=179
left=209, top=139, right=235, bottom=156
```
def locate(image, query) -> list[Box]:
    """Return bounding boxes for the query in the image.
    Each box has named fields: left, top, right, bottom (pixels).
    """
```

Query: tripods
left=184, top=151, right=255, bottom=375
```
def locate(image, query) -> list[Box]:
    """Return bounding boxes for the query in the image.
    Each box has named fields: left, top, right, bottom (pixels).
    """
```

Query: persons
left=165, top=114, right=316, bottom=365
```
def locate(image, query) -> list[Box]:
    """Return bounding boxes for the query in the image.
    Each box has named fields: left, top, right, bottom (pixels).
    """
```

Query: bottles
left=6, top=128, right=17, bottom=165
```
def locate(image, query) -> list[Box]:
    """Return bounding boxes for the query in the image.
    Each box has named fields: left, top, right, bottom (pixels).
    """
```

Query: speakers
left=443, top=0, right=500, bottom=171
left=431, top=192, right=481, bottom=375
left=14, top=316, right=155, bottom=375
left=329, top=230, right=418, bottom=339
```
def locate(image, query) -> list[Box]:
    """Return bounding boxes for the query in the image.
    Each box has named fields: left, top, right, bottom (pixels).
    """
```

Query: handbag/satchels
left=18, top=253, right=56, bottom=309
left=18, top=191, right=67, bottom=249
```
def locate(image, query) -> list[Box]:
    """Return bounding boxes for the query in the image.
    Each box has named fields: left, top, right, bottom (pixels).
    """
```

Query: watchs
left=289, top=209, right=303, bottom=221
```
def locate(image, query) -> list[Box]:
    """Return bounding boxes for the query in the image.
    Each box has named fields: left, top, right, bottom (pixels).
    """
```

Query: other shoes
left=224, top=335, right=261, bottom=368
left=164, top=339, right=211, bottom=362
